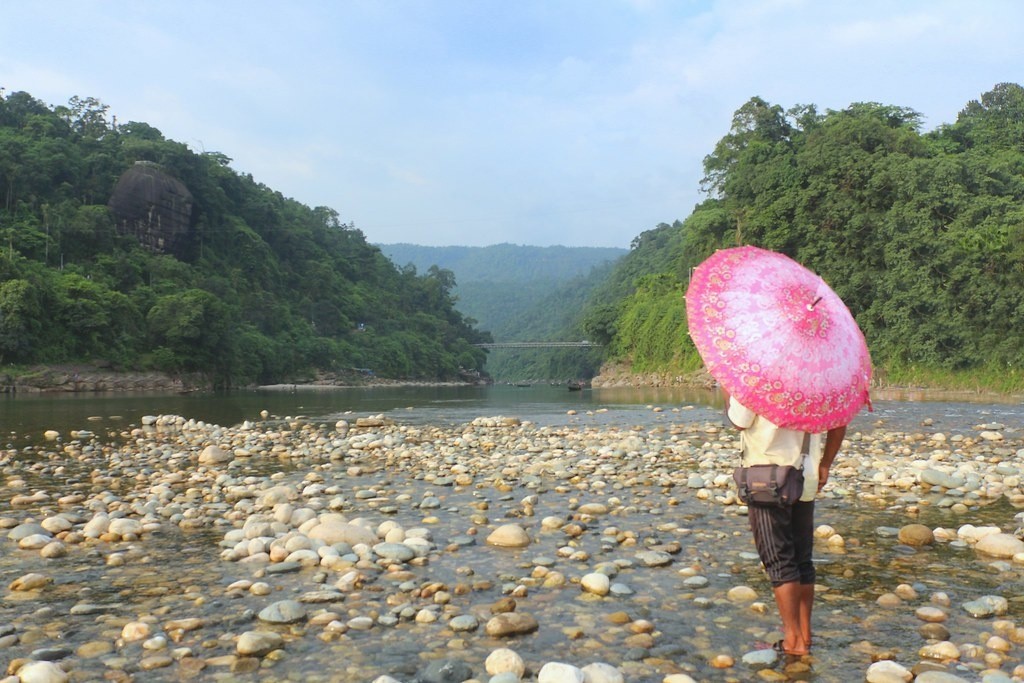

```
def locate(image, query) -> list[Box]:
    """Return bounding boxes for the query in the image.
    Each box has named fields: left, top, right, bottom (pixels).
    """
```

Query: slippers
left=772, top=640, right=809, bottom=656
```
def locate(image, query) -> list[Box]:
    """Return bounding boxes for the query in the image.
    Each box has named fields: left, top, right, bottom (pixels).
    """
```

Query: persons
left=719, top=382, right=848, bottom=663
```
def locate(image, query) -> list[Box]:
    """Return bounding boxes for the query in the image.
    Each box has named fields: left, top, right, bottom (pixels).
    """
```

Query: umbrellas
left=683, top=245, right=872, bottom=432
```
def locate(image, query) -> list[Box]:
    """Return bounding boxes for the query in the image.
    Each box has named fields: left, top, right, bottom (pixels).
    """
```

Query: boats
left=505, top=382, right=516, bottom=386
left=569, top=382, right=584, bottom=390
left=551, top=380, right=561, bottom=386
left=517, top=383, right=530, bottom=387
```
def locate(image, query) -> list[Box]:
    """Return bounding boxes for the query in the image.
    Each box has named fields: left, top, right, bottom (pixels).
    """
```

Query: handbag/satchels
left=733, top=464, right=805, bottom=506
